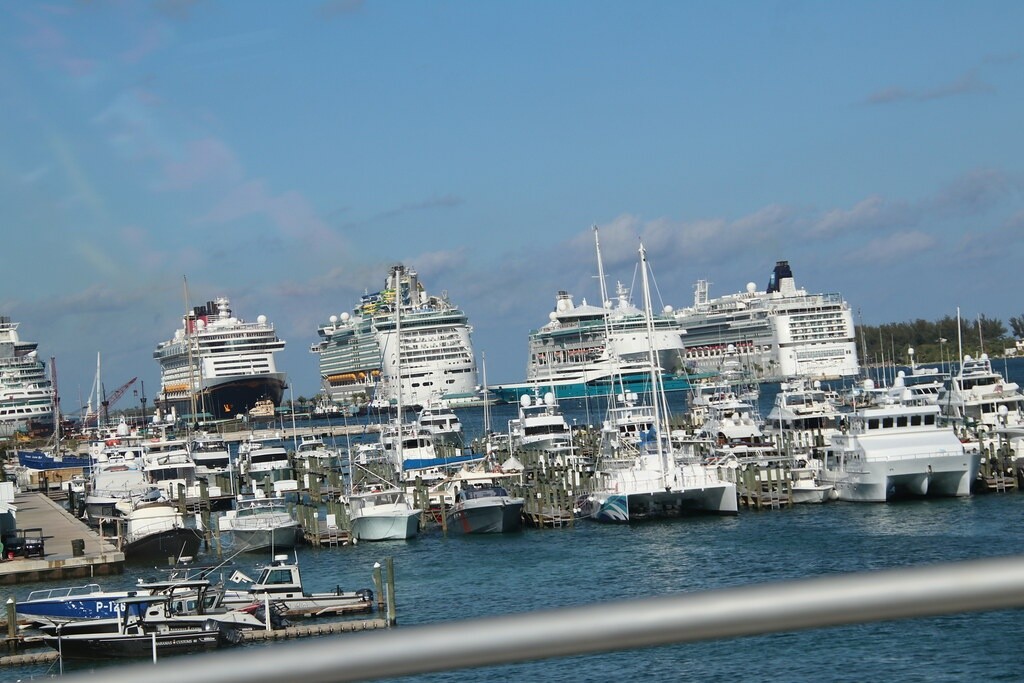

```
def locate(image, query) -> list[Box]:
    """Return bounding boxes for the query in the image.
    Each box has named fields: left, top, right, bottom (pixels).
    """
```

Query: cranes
left=83, top=377, right=140, bottom=429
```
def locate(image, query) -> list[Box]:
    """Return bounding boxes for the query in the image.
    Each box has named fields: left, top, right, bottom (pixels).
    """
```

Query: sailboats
left=0, top=221, right=1024, bottom=662
